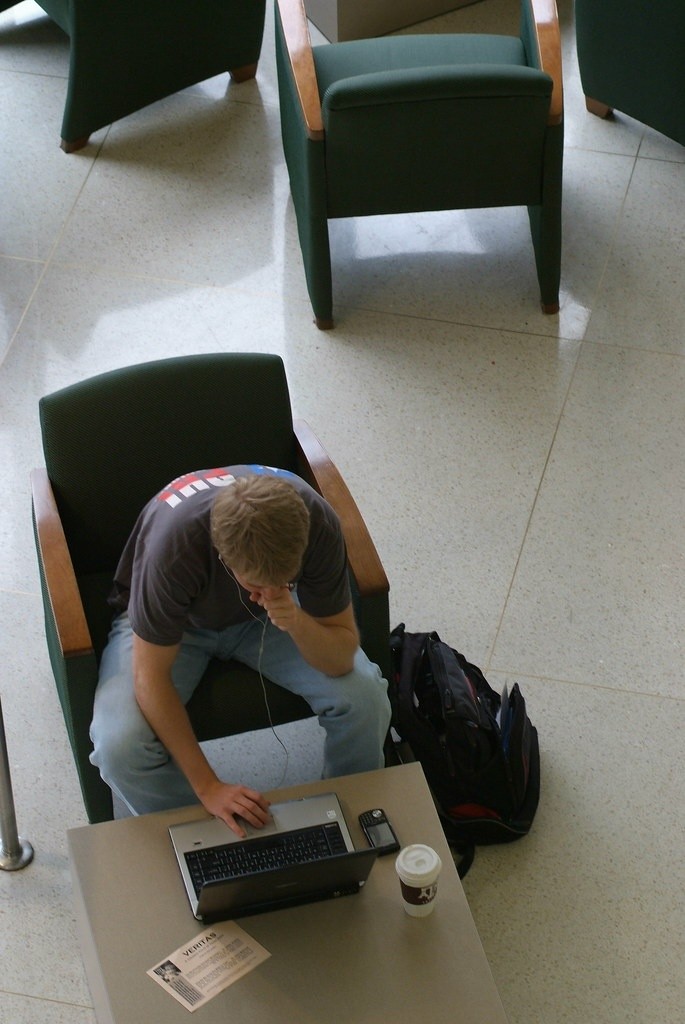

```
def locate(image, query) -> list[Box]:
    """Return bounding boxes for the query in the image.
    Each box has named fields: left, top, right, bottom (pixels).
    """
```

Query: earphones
left=219, top=553, right=221, bottom=560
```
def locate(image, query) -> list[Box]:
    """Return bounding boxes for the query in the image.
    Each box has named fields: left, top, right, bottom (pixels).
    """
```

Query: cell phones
left=358, top=809, right=400, bottom=857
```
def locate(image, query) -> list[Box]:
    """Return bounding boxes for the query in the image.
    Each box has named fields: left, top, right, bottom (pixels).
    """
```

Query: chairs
left=31, top=352, right=391, bottom=824
left=0, top=0, right=685, bottom=334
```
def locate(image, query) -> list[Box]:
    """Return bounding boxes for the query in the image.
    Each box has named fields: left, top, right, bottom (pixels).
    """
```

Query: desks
left=65, top=760, right=513, bottom=1023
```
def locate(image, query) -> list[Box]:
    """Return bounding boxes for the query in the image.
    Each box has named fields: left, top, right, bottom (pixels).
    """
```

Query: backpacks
left=378, top=623, right=542, bottom=882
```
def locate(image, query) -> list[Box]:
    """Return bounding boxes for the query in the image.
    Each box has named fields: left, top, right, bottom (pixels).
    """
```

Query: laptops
left=171, top=792, right=378, bottom=923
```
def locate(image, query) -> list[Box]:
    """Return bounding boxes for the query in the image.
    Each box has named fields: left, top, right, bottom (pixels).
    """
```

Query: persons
left=87, top=465, right=391, bottom=838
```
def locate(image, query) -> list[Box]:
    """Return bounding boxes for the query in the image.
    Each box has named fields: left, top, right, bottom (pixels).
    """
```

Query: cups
left=395, top=843, right=442, bottom=918
